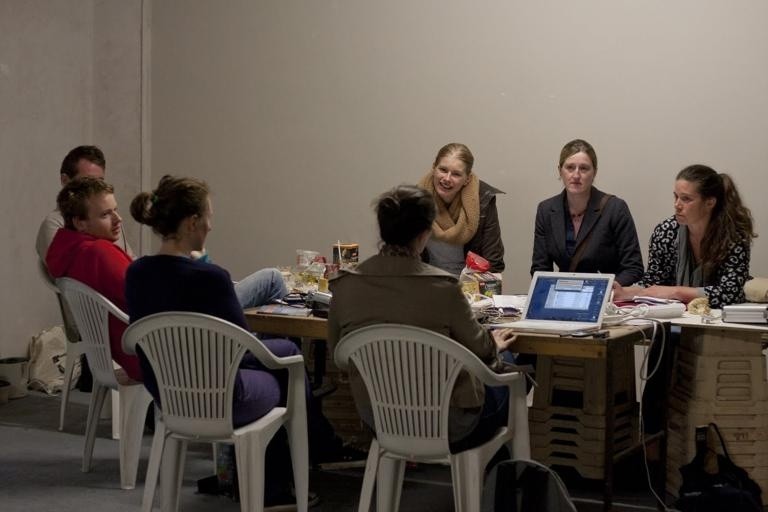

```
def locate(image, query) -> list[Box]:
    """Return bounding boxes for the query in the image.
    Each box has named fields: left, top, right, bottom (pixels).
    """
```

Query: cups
left=318, top=278, right=328, bottom=292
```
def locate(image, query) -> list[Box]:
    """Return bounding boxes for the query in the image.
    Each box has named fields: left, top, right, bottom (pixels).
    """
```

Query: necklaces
left=568, top=208, right=586, bottom=218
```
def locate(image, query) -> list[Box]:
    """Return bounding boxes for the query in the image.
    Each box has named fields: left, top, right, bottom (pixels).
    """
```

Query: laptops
left=494, top=271, right=615, bottom=332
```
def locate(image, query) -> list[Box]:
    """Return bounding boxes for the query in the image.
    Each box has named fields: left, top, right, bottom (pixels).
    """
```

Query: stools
left=531, top=355, right=640, bottom=479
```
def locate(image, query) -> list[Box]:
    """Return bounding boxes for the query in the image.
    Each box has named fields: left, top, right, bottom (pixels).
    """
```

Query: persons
left=506, top=138, right=646, bottom=397
left=45, top=175, right=314, bottom=384
left=612, top=163, right=759, bottom=494
left=327, top=181, right=536, bottom=474
left=122, top=172, right=369, bottom=511
left=35, top=144, right=139, bottom=270
left=412, top=141, right=505, bottom=279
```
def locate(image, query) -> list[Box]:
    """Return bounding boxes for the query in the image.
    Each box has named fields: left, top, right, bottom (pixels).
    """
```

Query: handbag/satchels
left=677, top=422, right=762, bottom=512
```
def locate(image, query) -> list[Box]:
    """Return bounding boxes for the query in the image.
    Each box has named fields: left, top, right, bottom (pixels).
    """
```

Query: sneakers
left=264, top=488, right=318, bottom=511
left=321, top=441, right=368, bottom=470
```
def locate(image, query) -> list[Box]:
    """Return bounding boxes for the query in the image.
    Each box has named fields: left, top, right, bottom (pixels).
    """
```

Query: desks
left=242, top=296, right=669, bottom=480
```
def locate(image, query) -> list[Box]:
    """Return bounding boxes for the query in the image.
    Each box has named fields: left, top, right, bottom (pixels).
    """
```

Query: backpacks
left=25, top=324, right=82, bottom=396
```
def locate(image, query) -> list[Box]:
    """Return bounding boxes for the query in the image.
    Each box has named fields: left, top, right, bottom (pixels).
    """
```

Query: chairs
left=122, top=310, right=309, bottom=512
left=53, top=277, right=160, bottom=489
left=33, top=258, right=90, bottom=431
left=332, top=322, right=531, bottom=512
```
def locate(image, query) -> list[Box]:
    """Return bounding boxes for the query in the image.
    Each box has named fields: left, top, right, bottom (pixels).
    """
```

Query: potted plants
left=0, top=375, right=12, bottom=404
